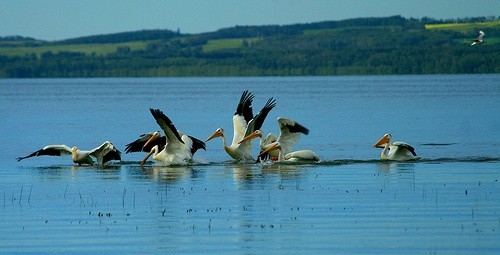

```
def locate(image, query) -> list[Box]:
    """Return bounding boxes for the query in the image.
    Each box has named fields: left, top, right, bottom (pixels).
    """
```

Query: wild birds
left=470, top=30, right=486, bottom=46
left=16, top=141, right=122, bottom=168
left=125, top=109, right=207, bottom=167
left=206, top=90, right=320, bottom=166
left=374, top=134, right=421, bottom=162
